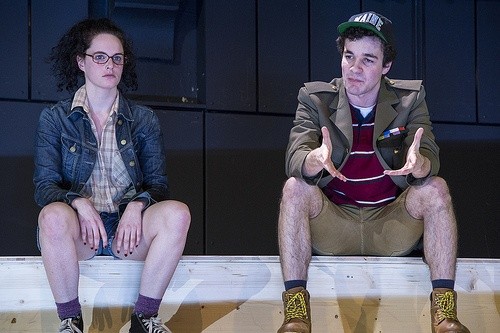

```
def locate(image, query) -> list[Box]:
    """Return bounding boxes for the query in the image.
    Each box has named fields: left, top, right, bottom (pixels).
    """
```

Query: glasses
left=83, top=51, right=128, bottom=65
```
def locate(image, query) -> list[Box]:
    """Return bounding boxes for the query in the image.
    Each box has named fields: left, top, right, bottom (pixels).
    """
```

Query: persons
left=278, top=12, right=471, bottom=333
left=34, top=16, right=190, bottom=333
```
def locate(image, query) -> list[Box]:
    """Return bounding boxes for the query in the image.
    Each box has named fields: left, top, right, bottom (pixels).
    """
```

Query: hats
left=337, top=11, right=392, bottom=44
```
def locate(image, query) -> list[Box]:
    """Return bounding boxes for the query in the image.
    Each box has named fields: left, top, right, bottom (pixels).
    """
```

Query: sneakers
left=129, top=312, right=171, bottom=333
left=58, top=311, right=83, bottom=333
left=277, top=287, right=311, bottom=333
left=431, top=288, right=471, bottom=333
